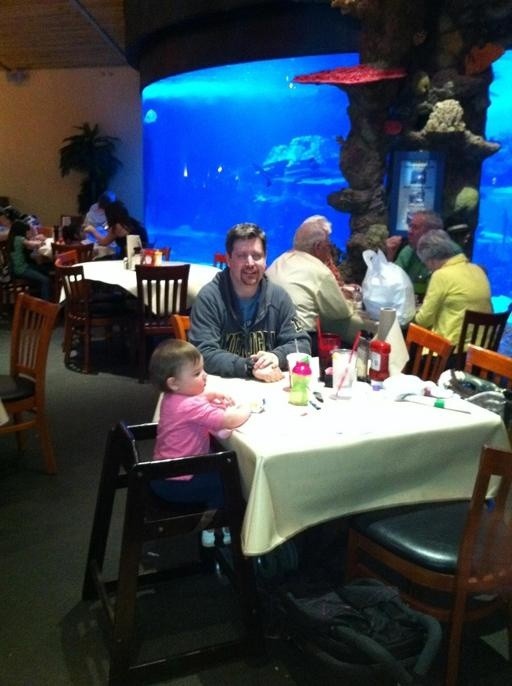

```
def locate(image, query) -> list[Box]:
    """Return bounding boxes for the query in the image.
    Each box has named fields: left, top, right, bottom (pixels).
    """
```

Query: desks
left=60, top=260, right=224, bottom=316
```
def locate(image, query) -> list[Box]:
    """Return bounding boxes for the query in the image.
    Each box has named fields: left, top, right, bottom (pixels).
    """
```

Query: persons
left=264, top=221, right=364, bottom=356
left=188, top=223, right=312, bottom=383
left=385, top=210, right=463, bottom=304
left=147, top=338, right=251, bottom=548
left=413, top=229, right=494, bottom=379
left=0, top=190, right=148, bottom=298
left=304, top=214, right=363, bottom=300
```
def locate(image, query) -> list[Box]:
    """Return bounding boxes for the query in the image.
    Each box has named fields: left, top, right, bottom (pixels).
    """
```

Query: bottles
left=351, top=288, right=361, bottom=311
left=290, top=361, right=309, bottom=404
left=368, top=340, right=391, bottom=381
left=132, top=247, right=162, bottom=271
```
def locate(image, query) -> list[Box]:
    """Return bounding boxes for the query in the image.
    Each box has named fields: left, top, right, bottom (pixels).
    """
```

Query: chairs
left=399, top=321, right=453, bottom=384
left=462, top=341, right=512, bottom=419
left=56, top=265, right=130, bottom=373
left=1, top=291, right=59, bottom=467
left=457, top=303, right=511, bottom=357
left=135, top=265, right=188, bottom=374
left=78, top=414, right=270, bottom=686
left=3, top=226, right=171, bottom=314
left=213, top=252, right=228, bottom=269
left=339, top=445, right=510, bottom=684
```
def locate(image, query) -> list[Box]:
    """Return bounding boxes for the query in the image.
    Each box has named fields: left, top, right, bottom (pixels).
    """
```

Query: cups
left=332, top=349, right=357, bottom=400
left=316, top=333, right=340, bottom=380
left=285, top=353, right=310, bottom=372
left=325, top=350, right=334, bottom=387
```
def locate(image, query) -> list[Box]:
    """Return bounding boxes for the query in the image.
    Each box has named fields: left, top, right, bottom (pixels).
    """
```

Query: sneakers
left=201, top=531, right=232, bottom=547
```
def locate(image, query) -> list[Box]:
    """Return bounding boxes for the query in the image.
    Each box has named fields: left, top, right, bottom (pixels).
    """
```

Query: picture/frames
left=382, top=150, right=447, bottom=241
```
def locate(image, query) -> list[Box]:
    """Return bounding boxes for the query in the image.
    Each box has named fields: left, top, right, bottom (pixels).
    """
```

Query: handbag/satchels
left=437, top=369, right=506, bottom=418
